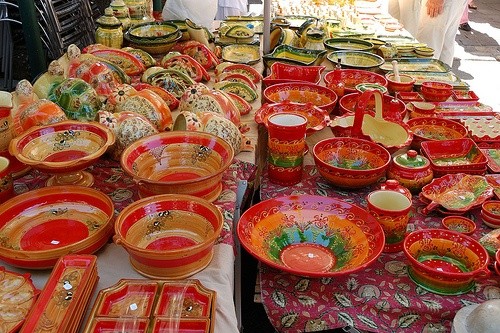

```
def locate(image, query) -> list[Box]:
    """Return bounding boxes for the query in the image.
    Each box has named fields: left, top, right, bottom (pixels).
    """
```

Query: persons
left=161, top=0, right=217, bottom=32
left=385, top=0, right=477, bottom=68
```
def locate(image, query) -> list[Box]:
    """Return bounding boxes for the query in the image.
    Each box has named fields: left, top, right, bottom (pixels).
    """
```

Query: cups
left=367, top=190, right=413, bottom=252
left=0, top=157, right=16, bottom=203
left=267, top=113, right=308, bottom=185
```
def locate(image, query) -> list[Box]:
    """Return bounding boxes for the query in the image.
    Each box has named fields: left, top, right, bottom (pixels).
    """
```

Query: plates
left=83, top=278, right=217, bottom=333
left=0, top=266, right=41, bottom=333
left=124, top=16, right=262, bottom=63
left=20, top=255, right=100, bottom=333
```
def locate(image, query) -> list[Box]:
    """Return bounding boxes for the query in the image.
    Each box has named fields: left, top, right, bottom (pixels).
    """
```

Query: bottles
left=387, top=150, right=436, bottom=192
left=0, top=0, right=264, bottom=155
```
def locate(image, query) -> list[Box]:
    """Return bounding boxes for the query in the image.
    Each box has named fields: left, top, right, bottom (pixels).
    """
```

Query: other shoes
left=460, top=24, right=471, bottom=31
left=469, top=4, right=477, bottom=9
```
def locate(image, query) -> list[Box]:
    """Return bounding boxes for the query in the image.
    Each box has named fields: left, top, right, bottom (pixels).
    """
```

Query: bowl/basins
left=0, top=120, right=235, bottom=281
left=237, top=0, right=500, bottom=333
